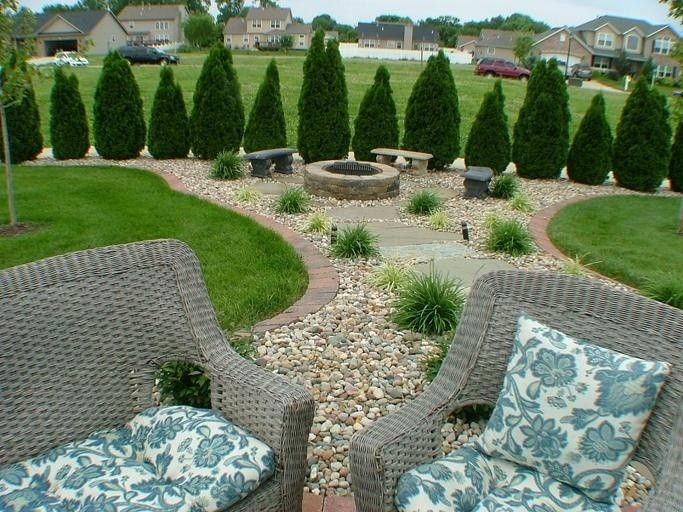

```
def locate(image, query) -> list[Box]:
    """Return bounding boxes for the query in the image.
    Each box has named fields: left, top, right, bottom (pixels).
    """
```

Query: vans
left=546, top=58, right=572, bottom=79
left=117, top=45, right=179, bottom=66
left=572, top=63, right=592, bottom=81
left=474, top=56, right=532, bottom=81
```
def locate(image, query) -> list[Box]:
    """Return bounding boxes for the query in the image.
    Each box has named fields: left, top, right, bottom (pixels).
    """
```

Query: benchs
left=243, top=147, right=298, bottom=177
left=370, top=148, right=433, bottom=177
left=460, top=165, right=493, bottom=199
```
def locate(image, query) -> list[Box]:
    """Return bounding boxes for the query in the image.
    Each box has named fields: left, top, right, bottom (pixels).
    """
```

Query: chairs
left=0, top=239, right=314, bottom=512
left=350, top=271, right=683, bottom=512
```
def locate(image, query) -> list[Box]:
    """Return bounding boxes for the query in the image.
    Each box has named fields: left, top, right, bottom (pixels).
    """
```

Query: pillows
left=475, top=311, right=673, bottom=505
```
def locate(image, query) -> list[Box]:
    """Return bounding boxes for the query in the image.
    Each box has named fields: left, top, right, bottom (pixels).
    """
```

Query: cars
left=53, top=50, right=89, bottom=67
